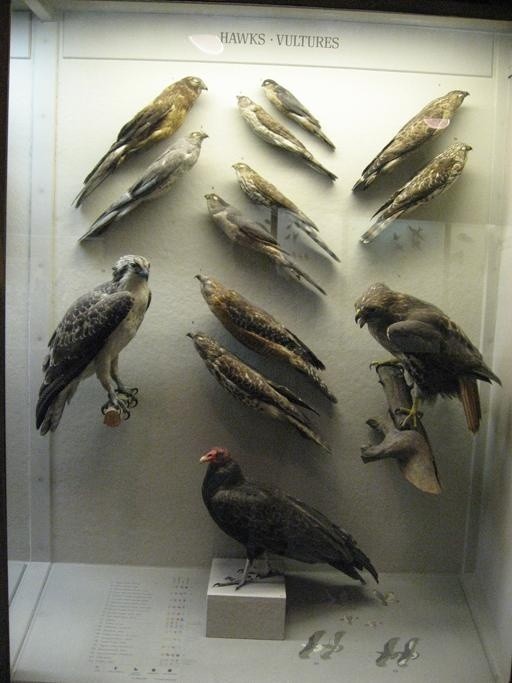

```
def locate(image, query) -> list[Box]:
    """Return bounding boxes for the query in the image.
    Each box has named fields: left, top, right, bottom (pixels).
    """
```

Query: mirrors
left=197, top=445, right=379, bottom=593
left=352, top=279, right=502, bottom=436
left=350, top=88, right=470, bottom=194
left=70, top=74, right=208, bottom=209
left=205, top=192, right=330, bottom=297
left=76, top=127, right=210, bottom=245
left=237, top=96, right=338, bottom=186
left=357, top=141, right=472, bottom=245
left=231, top=160, right=343, bottom=265
left=185, top=330, right=335, bottom=457
left=192, top=273, right=339, bottom=404
left=35, top=253, right=153, bottom=437
left=260, top=78, right=337, bottom=151
